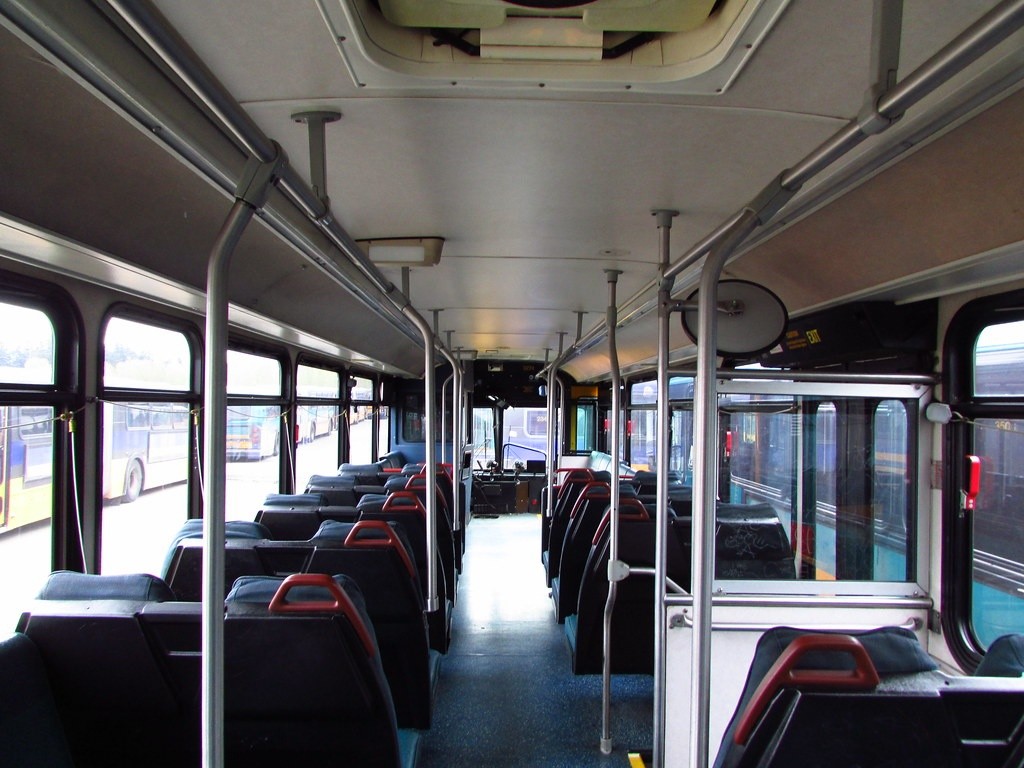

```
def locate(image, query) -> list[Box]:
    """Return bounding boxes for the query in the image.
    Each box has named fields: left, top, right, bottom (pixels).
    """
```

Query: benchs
left=0, top=452, right=465, bottom=768
left=713, top=627, right=1024, bottom=768
left=542, top=450, right=796, bottom=677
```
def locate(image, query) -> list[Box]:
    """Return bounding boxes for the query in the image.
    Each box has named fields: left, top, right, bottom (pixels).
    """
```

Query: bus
left=0, top=364, right=191, bottom=536
left=572, top=340, right=1024, bottom=650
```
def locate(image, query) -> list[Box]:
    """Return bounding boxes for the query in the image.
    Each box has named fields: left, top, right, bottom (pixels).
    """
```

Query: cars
left=224, top=383, right=388, bottom=465
